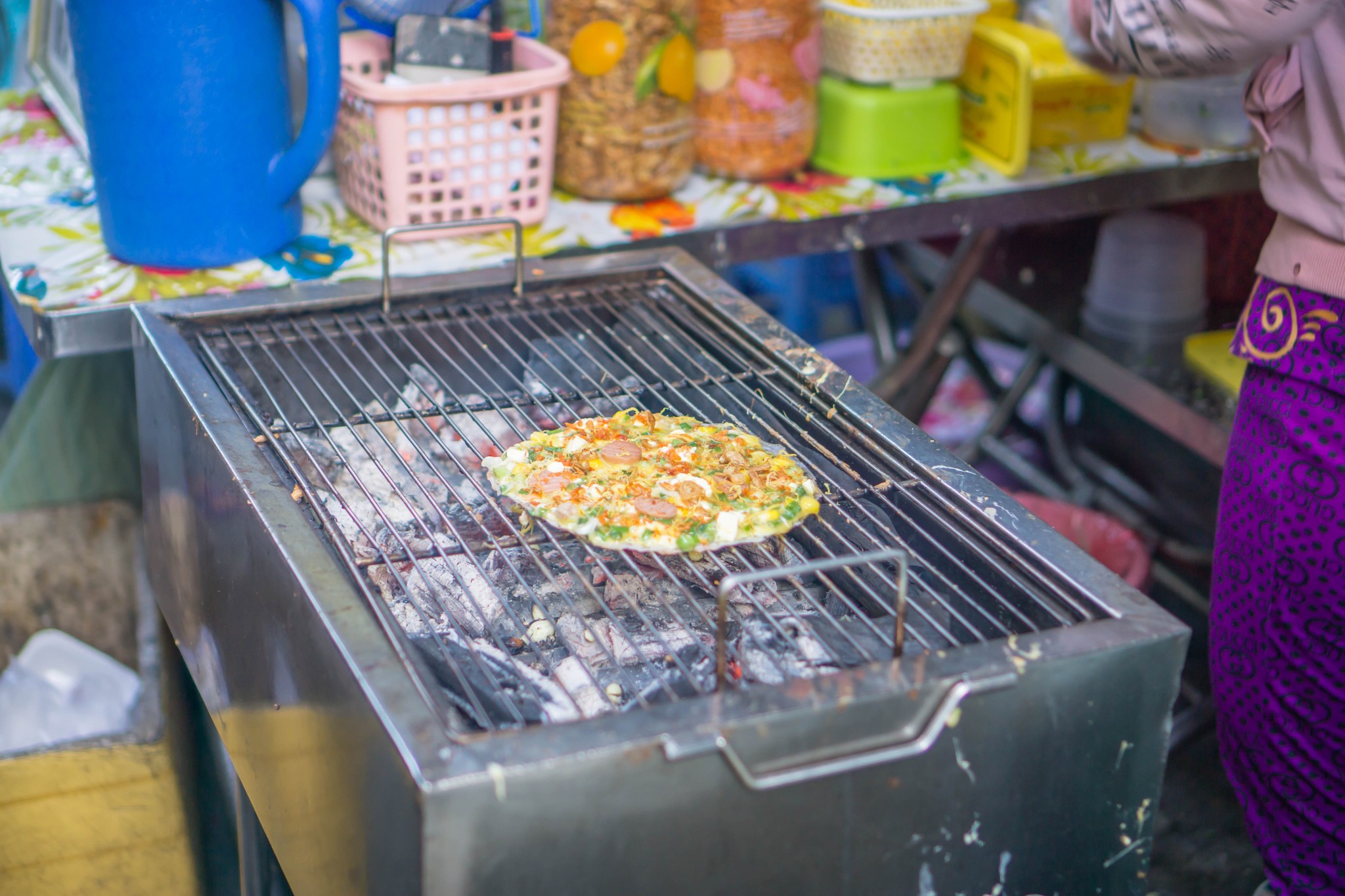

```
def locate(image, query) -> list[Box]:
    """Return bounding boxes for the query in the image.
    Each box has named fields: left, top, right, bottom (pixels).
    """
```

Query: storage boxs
left=0, top=493, right=204, bottom=896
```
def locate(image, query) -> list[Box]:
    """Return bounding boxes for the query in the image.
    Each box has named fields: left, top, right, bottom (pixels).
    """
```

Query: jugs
left=63, top=3, right=349, bottom=276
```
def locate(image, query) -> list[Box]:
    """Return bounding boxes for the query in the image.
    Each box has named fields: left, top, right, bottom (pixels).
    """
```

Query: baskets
left=301, top=24, right=570, bottom=240
left=820, top=2, right=985, bottom=82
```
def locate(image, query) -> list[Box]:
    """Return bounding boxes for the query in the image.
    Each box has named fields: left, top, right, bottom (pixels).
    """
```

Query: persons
left=1091, top=0, right=1345, bottom=896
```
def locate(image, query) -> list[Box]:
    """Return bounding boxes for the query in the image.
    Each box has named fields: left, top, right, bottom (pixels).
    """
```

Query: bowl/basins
left=1081, top=210, right=1207, bottom=321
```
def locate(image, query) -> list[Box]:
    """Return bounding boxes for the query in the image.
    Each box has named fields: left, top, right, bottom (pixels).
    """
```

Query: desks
left=895, top=213, right=1249, bottom=756
left=3, top=60, right=1264, bottom=452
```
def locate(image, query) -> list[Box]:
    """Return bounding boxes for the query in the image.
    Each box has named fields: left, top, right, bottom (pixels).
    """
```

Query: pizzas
left=484, top=405, right=822, bottom=555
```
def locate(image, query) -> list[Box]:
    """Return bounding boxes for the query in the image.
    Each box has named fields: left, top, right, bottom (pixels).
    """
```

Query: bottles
left=698, top=0, right=819, bottom=177
left=533, top=1, right=703, bottom=203
